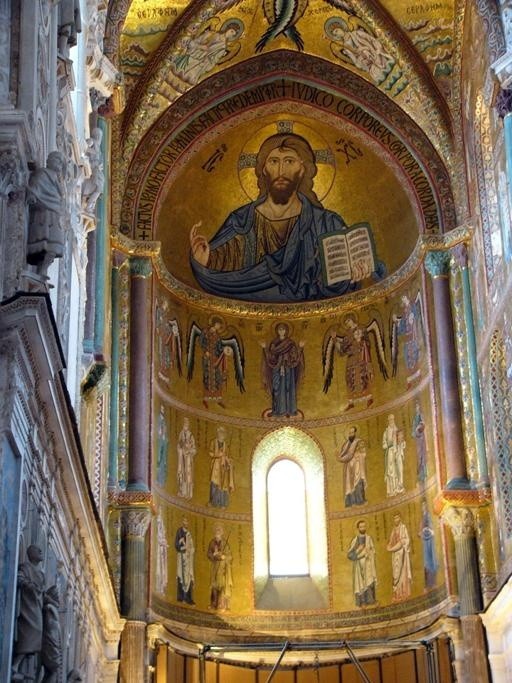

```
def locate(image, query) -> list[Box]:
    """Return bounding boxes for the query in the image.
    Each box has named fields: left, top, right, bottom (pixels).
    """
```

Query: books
left=317, top=222, right=379, bottom=287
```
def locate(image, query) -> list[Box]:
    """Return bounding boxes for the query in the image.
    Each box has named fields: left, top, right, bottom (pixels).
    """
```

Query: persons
left=176, top=416, right=197, bottom=500
left=206, top=425, right=235, bottom=511
left=82, top=127, right=105, bottom=213
left=416, top=497, right=438, bottom=594
left=388, top=288, right=427, bottom=377
left=346, top=520, right=378, bottom=608
left=335, top=426, right=368, bottom=508
left=174, top=517, right=197, bottom=606
left=189, top=130, right=388, bottom=304
left=261, top=322, right=305, bottom=418
left=154, top=297, right=184, bottom=391
left=27, top=151, right=67, bottom=289
left=385, top=509, right=414, bottom=604
left=155, top=400, right=170, bottom=488
left=157, top=504, right=170, bottom=594
left=328, top=21, right=393, bottom=81
left=410, top=398, right=428, bottom=485
left=11, top=544, right=48, bottom=680
left=320, top=308, right=389, bottom=413
left=207, top=525, right=234, bottom=611
left=185, top=313, right=247, bottom=409
left=382, top=413, right=406, bottom=498
left=173, top=21, right=241, bottom=83
left=40, top=584, right=63, bottom=683
